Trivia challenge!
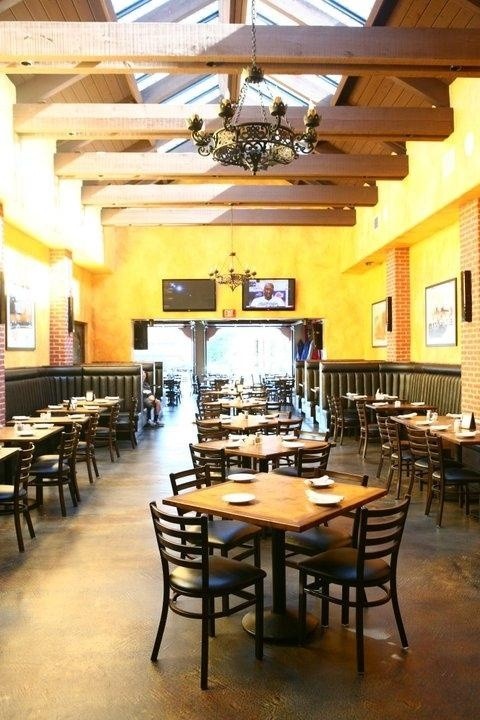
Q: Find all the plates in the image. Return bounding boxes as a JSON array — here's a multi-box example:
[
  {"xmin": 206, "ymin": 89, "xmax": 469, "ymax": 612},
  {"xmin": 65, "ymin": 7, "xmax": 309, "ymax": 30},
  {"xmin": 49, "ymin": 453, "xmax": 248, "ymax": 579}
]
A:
[
  {"xmin": 219, "ymin": 414, "xmax": 277, "ymax": 424},
  {"xmin": 12, "ymin": 415, "xmax": 30, "ymax": 420},
  {"xmin": 48, "ymin": 405, "xmax": 63, "ymax": 410},
  {"xmin": 69, "ymin": 413, "xmax": 86, "ymax": 419},
  {"xmin": 85, "ymin": 405, "xmax": 99, "ymax": 409},
  {"xmin": 398, "ymin": 412, "xmax": 476, "ymax": 439},
  {"xmin": 226, "ymin": 435, "xmax": 304, "ymax": 450},
  {"xmin": 410, "ymin": 402, "xmax": 426, "ymax": 405},
  {"xmin": 32, "ymin": 424, "xmax": 54, "ymax": 428},
  {"xmin": 373, "ymin": 402, "xmax": 388, "ymax": 406},
  {"xmin": 226, "ymin": 473, "xmax": 344, "ymax": 524}
]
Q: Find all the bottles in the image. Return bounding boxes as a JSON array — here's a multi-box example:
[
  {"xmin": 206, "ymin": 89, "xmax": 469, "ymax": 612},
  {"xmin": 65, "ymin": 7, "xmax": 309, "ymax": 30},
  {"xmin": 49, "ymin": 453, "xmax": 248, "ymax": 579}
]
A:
[
  {"xmin": 244, "ymin": 412, "xmax": 248, "ymax": 420},
  {"xmin": 40, "ymin": 413, "xmax": 46, "ymax": 421},
  {"xmin": 47, "ymin": 411, "xmax": 51, "ymax": 418},
  {"xmin": 256, "ymin": 433, "xmax": 261, "ymax": 444}
]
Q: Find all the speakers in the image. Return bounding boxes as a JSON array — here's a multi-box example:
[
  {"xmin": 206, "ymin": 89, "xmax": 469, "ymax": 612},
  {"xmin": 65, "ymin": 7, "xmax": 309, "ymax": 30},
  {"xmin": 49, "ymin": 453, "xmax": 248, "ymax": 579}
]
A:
[
  {"xmin": 133, "ymin": 321, "xmax": 148, "ymax": 350},
  {"xmin": 312, "ymin": 321, "xmax": 323, "ymax": 350}
]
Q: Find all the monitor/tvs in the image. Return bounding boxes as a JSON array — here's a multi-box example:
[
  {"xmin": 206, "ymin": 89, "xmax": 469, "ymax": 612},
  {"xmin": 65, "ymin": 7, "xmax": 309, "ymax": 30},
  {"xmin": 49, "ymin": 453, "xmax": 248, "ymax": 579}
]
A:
[
  {"xmin": 162, "ymin": 278, "xmax": 216, "ymax": 311},
  {"xmin": 242, "ymin": 278, "xmax": 296, "ymax": 311}
]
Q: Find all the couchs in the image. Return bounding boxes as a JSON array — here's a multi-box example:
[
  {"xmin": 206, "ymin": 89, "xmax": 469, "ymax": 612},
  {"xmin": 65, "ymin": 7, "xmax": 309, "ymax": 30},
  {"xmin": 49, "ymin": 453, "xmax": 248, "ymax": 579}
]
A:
[
  {"xmin": 320, "ymin": 360, "xmax": 461, "ymax": 434},
  {"xmin": 156, "ymin": 362, "xmax": 163, "ymax": 409},
  {"xmin": 5, "ymin": 366, "xmax": 141, "ymax": 440},
  {"xmin": 295, "ymin": 361, "xmax": 303, "ymax": 413},
  {"xmin": 144, "ymin": 363, "xmax": 154, "ymax": 426},
  {"xmin": 305, "ymin": 362, "xmax": 319, "ymax": 423}
]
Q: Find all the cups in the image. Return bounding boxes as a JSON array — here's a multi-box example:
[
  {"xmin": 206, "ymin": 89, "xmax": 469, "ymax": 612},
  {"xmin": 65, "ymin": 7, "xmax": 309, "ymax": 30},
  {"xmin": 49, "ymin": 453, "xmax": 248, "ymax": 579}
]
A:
[{"xmin": 395, "ymin": 401, "xmax": 401, "ymax": 408}]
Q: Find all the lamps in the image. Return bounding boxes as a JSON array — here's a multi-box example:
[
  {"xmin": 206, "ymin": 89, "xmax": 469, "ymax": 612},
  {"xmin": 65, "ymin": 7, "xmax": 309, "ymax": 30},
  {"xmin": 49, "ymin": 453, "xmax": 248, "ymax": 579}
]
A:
[
  {"xmin": 187, "ymin": 1, "xmax": 323, "ymax": 178},
  {"xmin": 209, "ymin": 204, "xmax": 261, "ymax": 291}
]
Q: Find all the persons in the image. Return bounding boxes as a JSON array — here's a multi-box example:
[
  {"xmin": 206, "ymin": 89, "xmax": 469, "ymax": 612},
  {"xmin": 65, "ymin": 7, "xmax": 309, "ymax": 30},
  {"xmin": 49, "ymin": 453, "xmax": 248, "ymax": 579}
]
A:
[
  {"xmin": 140, "ymin": 370, "xmax": 165, "ymax": 428},
  {"xmin": 250, "ymin": 283, "xmax": 285, "ymax": 308}
]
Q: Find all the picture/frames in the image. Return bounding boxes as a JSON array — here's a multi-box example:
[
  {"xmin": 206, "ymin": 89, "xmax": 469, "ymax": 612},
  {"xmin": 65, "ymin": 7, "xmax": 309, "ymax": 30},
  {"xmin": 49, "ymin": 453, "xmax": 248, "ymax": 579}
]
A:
[
  {"xmin": 372, "ymin": 300, "xmax": 388, "ymax": 348},
  {"xmin": 424, "ymin": 278, "xmax": 458, "ymax": 349},
  {"xmin": 5, "ymin": 278, "xmax": 36, "ymax": 352}
]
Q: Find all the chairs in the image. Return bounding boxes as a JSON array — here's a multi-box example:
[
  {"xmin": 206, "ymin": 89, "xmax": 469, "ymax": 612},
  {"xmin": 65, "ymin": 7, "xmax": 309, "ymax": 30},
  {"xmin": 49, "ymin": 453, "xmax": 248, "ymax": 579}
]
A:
[
  {"xmin": 297, "ymin": 443, "xmax": 331, "ymax": 527},
  {"xmin": 285, "ymin": 468, "xmax": 369, "ymax": 627},
  {"xmin": 169, "ymin": 464, "xmax": 262, "ymax": 568},
  {"xmin": 57, "ymin": 414, "xmax": 100, "ymax": 483},
  {"xmin": 92, "ymin": 402, "xmax": 120, "ymax": 462},
  {"xmin": 21, "ymin": 428, "xmax": 78, "ymax": 516},
  {"xmin": 112, "ymin": 394, "xmax": 138, "ymax": 449},
  {"xmin": 328, "ymin": 395, "xmax": 480, "ymax": 528},
  {"xmin": 298, "ymin": 495, "xmax": 411, "ymax": 675},
  {"xmin": 189, "ymin": 443, "xmax": 226, "ymax": 516},
  {"xmin": 149, "ymin": 501, "xmax": 266, "ymax": 691},
  {"xmin": 195, "ymin": 371, "xmax": 302, "ymax": 439},
  {"xmin": 0, "ymin": 442, "xmax": 35, "ymax": 553}
]
